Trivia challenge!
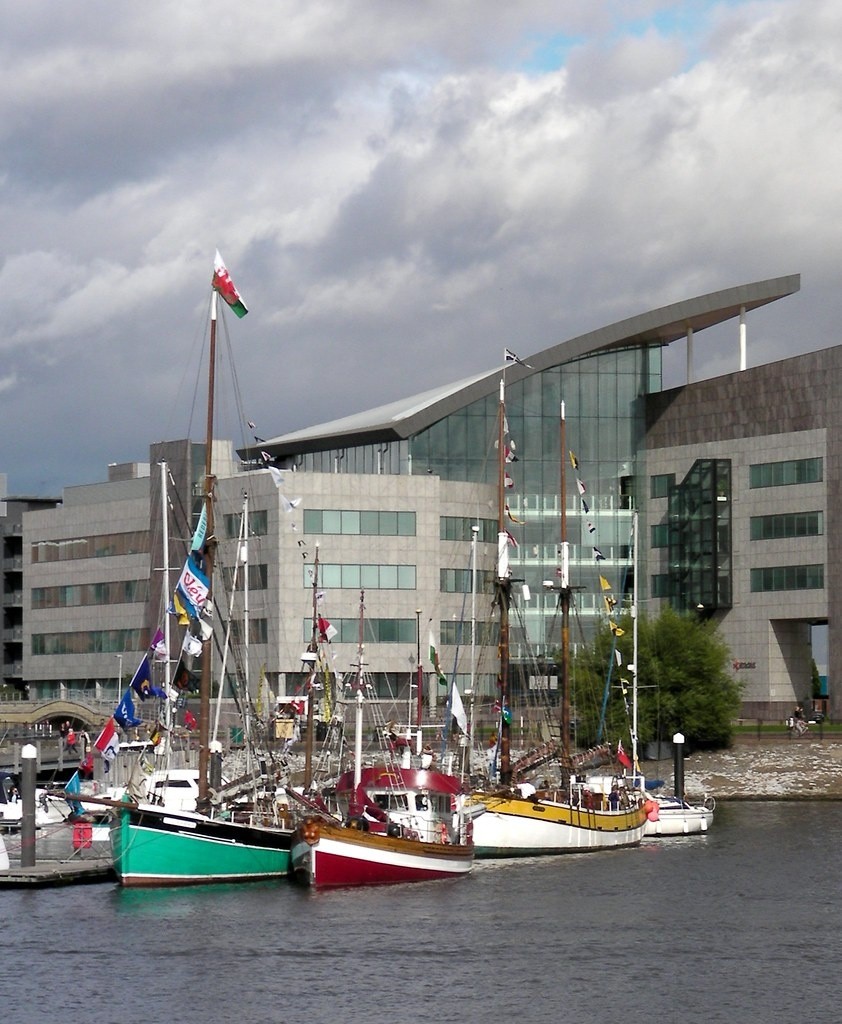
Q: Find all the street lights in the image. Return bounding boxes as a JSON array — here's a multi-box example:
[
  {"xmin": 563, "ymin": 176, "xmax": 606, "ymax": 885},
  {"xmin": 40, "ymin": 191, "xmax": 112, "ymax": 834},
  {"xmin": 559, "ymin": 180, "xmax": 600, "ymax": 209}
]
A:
[
  {"xmin": 114, "ymin": 654, "xmax": 123, "ymax": 704},
  {"xmin": 415, "ymin": 609, "xmax": 423, "ymax": 666}
]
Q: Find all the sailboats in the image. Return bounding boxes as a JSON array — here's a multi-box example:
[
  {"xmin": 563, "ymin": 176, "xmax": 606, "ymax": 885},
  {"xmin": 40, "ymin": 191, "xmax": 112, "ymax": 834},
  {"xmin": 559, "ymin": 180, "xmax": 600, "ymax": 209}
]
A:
[{"xmin": 0, "ymin": 249, "xmax": 715, "ymax": 887}]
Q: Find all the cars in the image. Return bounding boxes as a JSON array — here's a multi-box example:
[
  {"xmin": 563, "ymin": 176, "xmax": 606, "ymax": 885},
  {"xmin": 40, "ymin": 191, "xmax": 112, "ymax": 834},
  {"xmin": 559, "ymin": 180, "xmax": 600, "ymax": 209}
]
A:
[{"xmin": 811, "ymin": 712, "xmax": 825, "ymax": 724}]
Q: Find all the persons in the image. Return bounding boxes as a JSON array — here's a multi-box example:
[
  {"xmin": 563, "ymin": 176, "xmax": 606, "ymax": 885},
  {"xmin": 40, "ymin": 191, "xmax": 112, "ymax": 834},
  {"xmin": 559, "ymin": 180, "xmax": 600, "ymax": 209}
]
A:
[
  {"xmin": 514, "ymin": 778, "xmax": 536, "ymax": 800},
  {"xmin": 793, "ymin": 706, "xmax": 804, "ymax": 721},
  {"xmin": 9, "ymin": 786, "xmax": 19, "ymax": 804},
  {"xmin": 608, "ymin": 785, "xmax": 629, "ymax": 811},
  {"xmin": 100, "ymin": 719, "xmax": 105, "ymax": 726},
  {"xmin": 61, "ymin": 721, "xmax": 91, "ymax": 755},
  {"xmin": 539, "ymin": 780, "xmax": 550, "ymax": 789}
]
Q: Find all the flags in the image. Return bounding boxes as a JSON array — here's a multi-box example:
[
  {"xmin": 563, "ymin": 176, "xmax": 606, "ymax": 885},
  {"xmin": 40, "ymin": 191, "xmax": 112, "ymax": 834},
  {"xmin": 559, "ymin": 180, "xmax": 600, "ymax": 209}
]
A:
[
  {"xmin": 181, "ymin": 629, "xmax": 203, "ymax": 657},
  {"xmin": 62, "ymin": 771, "xmax": 86, "ymax": 819},
  {"xmin": 171, "ymin": 555, "xmax": 212, "ymax": 624},
  {"xmin": 150, "ymin": 730, "xmax": 161, "ymax": 746},
  {"xmin": 173, "ymin": 657, "xmax": 202, "ymax": 693},
  {"xmin": 445, "ymin": 681, "xmax": 468, "ymax": 737},
  {"xmin": 112, "ymin": 689, "xmax": 144, "ymax": 731},
  {"xmin": 130, "ymin": 657, "xmax": 168, "ymax": 703},
  {"xmin": 429, "ymin": 629, "xmax": 448, "ymax": 687},
  {"xmin": 150, "ymin": 629, "xmax": 166, "ymax": 656},
  {"xmin": 190, "ymin": 615, "xmax": 214, "ymax": 640},
  {"xmin": 184, "ymin": 709, "xmax": 197, "ymax": 731},
  {"xmin": 95, "ymin": 717, "xmax": 120, "ymax": 763},
  {"xmin": 318, "ymin": 616, "xmax": 338, "ymax": 642},
  {"xmin": 618, "ymin": 742, "xmax": 631, "ymax": 772},
  {"xmin": 212, "ymin": 248, "xmax": 248, "ymax": 319}
]
